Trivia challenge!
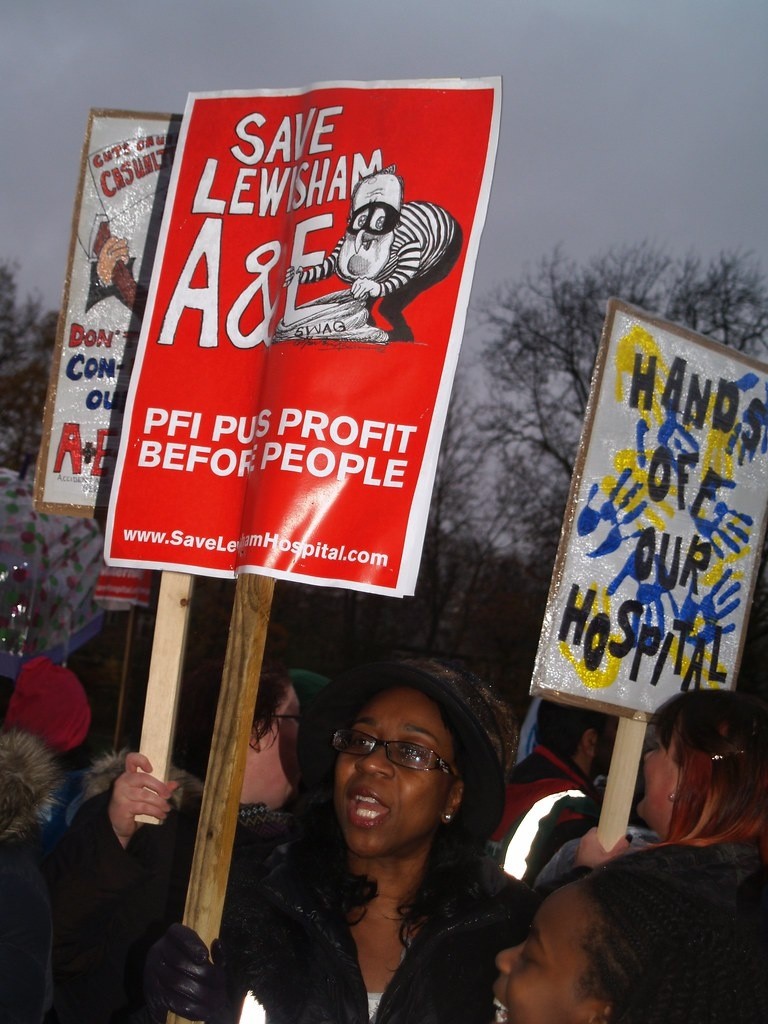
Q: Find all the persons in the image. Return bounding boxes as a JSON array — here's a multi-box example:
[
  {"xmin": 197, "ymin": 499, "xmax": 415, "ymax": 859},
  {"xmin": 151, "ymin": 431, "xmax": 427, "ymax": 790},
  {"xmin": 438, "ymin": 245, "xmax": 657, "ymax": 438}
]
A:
[{"xmin": 0, "ymin": 623, "xmax": 768, "ymax": 1024}]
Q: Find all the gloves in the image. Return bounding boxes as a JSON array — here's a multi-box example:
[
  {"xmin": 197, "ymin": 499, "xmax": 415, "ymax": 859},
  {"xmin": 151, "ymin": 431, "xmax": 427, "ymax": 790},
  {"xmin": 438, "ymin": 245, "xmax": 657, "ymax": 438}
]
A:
[{"xmin": 144, "ymin": 924, "xmax": 236, "ymax": 1024}]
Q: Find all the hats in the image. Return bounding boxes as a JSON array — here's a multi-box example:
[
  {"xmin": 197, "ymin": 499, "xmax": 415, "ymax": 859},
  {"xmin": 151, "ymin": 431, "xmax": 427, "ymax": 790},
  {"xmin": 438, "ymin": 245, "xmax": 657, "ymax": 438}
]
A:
[
  {"xmin": 297, "ymin": 657, "xmax": 518, "ymax": 837},
  {"xmin": 4, "ymin": 657, "xmax": 91, "ymax": 752},
  {"xmin": 289, "ymin": 668, "xmax": 333, "ymax": 714}
]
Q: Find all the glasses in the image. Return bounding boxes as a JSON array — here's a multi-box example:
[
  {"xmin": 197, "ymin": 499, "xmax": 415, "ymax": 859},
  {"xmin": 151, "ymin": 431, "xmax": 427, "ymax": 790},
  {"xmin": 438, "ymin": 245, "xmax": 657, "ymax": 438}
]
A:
[{"xmin": 333, "ymin": 728, "xmax": 459, "ymax": 778}]
